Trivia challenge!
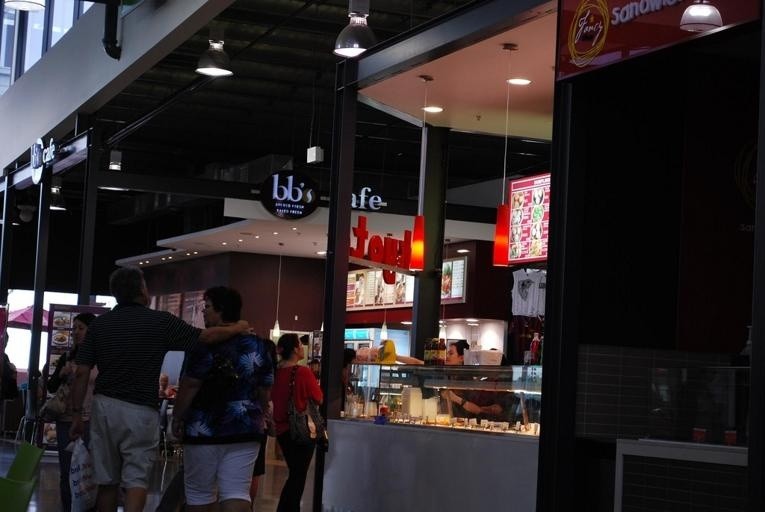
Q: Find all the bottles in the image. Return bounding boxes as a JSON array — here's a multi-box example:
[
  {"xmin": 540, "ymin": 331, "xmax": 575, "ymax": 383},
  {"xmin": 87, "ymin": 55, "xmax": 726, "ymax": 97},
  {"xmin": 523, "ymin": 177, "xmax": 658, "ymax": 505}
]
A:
[
  {"xmin": 530, "ymin": 332, "xmax": 544, "ymax": 365},
  {"xmin": 423, "ymin": 337, "xmax": 446, "ymax": 364}
]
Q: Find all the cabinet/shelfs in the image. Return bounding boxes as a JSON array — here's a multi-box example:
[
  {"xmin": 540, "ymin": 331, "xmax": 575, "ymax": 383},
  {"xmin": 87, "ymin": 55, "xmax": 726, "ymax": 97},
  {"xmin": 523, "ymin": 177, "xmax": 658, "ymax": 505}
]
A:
[{"xmin": 321, "ymin": 363, "xmax": 542, "ymax": 511}]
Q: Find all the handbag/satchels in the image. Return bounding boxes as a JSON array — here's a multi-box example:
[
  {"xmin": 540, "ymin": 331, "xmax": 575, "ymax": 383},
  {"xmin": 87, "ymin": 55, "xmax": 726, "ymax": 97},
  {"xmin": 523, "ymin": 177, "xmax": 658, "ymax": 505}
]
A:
[
  {"xmin": 38, "ymin": 386, "xmax": 70, "ymax": 424},
  {"xmin": 287, "ymin": 365, "xmax": 329, "ymax": 452}
]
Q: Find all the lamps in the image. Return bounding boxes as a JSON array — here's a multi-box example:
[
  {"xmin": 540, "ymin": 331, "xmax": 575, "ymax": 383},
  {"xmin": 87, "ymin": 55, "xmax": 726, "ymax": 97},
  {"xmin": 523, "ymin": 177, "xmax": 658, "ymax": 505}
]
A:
[
  {"xmin": 272, "ymin": 243, "xmax": 284, "ymax": 338},
  {"xmin": 409, "ymin": 72, "xmax": 434, "ymax": 271},
  {"xmin": 680, "ymin": 0, "xmax": 723, "ymax": 33},
  {"xmin": 332, "ymin": 0, "xmax": 376, "ymax": 58},
  {"xmin": 492, "ymin": 42, "xmax": 519, "ymax": 268},
  {"xmin": 194, "ymin": 27, "xmax": 235, "ymax": 78},
  {"xmin": 17, "ymin": 205, "xmax": 37, "ymax": 222},
  {"xmin": 109, "ymin": 136, "xmax": 123, "ymax": 171},
  {"xmin": 49, "ymin": 175, "xmax": 67, "ymax": 211}
]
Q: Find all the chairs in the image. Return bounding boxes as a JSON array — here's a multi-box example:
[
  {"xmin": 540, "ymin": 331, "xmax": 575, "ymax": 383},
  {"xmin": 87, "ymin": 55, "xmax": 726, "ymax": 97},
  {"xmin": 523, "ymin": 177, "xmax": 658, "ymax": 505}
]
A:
[{"xmin": 0, "ymin": 388, "xmax": 183, "ymax": 512}]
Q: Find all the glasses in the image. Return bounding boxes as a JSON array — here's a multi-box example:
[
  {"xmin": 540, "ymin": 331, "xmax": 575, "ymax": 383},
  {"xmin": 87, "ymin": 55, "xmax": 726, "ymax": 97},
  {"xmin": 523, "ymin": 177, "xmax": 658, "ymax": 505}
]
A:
[{"xmin": 202, "ymin": 303, "xmax": 214, "ymax": 310}]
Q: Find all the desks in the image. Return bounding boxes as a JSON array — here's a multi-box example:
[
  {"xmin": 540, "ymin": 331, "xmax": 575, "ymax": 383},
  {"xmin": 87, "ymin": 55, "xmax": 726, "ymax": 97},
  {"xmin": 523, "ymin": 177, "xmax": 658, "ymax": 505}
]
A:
[{"xmin": 614, "ymin": 436, "xmax": 749, "ymax": 511}]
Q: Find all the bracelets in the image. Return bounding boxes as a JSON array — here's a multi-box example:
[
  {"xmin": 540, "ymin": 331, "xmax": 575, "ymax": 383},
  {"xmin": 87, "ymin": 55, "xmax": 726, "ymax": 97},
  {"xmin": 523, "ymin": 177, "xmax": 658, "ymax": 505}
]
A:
[
  {"xmin": 459, "ymin": 398, "xmax": 468, "ymax": 407},
  {"xmin": 72, "ymin": 406, "xmax": 84, "ymax": 414}
]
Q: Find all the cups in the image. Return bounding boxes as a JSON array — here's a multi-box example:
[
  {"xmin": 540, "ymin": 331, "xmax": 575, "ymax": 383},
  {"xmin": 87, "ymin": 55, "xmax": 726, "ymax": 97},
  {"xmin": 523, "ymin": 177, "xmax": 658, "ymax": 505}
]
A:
[
  {"xmin": 524, "ymin": 350, "xmax": 530, "ymax": 363},
  {"xmin": 469, "ymin": 334, "xmax": 482, "ymax": 366}
]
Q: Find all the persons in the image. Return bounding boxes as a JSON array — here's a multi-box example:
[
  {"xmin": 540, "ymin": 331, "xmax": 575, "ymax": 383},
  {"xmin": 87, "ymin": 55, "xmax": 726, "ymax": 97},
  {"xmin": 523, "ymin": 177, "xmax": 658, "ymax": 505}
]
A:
[
  {"xmin": 64, "ymin": 263, "xmax": 256, "ymax": 511},
  {"xmin": 157, "ymin": 371, "xmax": 171, "ymax": 409},
  {"xmin": 342, "ymin": 346, "xmax": 366, "ymax": 418},
  {"xmin": 247, "ymin": 429, "xmax": 269, "ymax": 511},
  {"xmin": 267, "ymin": 332, "xmax": 325, "ymax": 512},
  {"xmin": 463, "ymin": 347, "xmax": 514, "ymax": 422},
  {"xmin": 0, "ymin": 332, "xmax": 18, "ymax": 446},
  {"xmin": 170, "ymin": 283, "xmax": 277, "ymax": 511},
  {"xmin": 307, "ymin": 342, "xmax": 322, "ymax": 362},
  {"xmin": 17, "ymin": 368, "xmax": 41, "ymax": 401},
  {"xmin": 48, "ymin": 311, "xmax": 97, "ymax": 512},
  {"xmin": 393, "ymin": 339, "xmax": 482, "ymax": 419}
]
